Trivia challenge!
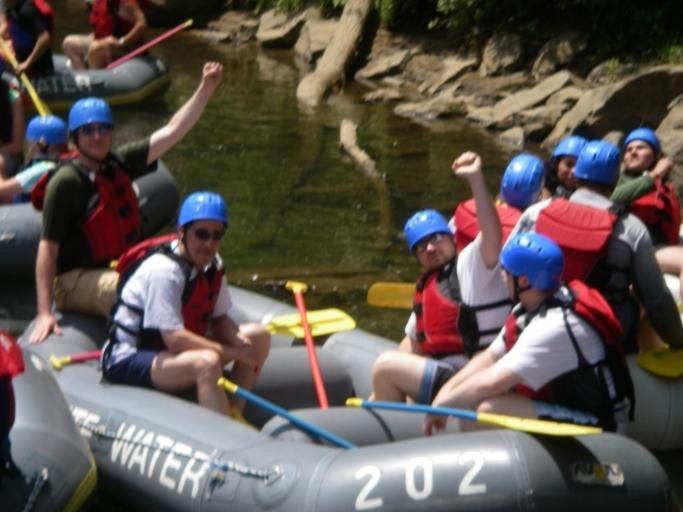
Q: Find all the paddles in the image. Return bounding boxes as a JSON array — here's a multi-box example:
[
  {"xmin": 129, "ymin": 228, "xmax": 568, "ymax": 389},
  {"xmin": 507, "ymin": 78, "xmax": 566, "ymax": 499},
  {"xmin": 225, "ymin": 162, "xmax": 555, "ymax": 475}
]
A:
[
  {"xmin": 50, "ymin": 308, "xmax": 356, "ymax": 370},
  {"xmin": 638, "ymin": 347, "xmax": 683, "ymax": 379},
  {"xmin": 347, "ymin": 396, "xmax": 604, "ymax": 436},
  {"xmin": 365, "ymin": 282, "xmax": 417, "ymax": 309},
  {"xmin": 2, "ymin": 37, "xmax": 53, "ymax": 118}
]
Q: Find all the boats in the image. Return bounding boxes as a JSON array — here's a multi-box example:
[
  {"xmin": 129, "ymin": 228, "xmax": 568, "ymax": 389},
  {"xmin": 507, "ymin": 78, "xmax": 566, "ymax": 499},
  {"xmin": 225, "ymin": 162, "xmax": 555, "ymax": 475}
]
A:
[
  {"xmin": 592, "ymin": 269, "xmax": 682, "ymax": 456},
  {"xmin": 0, "ymin": 333, "xmax": 98, "ymax": 511},
  {"xmin": 21, "ymin": 282, "xmax": 670, "ymax": 512},
  {"xmin": 0, "ymin": 152, "xmax": 180, "ymax": 271},
  {"xmin": 0, "ymin": 53, "xmax": 170, "ymax": 111}
]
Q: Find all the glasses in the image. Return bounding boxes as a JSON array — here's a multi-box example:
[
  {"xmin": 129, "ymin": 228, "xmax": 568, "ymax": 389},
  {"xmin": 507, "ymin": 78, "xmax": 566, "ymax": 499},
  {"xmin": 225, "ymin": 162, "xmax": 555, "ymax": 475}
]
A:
[
  {"xmin": 412, "ymin": 232, "xmax": 444, "ymax": 255},
  {"xmin": 80, "ymin": 124, "xmax": 112, "ymax": 136},
  {"xmin": 187, "ymin": 227, "xmax": 224, "ymax": 241}
]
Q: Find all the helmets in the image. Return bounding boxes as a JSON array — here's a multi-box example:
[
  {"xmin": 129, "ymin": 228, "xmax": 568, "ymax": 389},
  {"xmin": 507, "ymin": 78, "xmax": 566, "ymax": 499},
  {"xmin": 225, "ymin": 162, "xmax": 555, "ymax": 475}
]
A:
[
  {"xmin": 499, "ymin": 152, "xmax": 548, "ymax": 210},
  {"xmin": 66, "ymin": 95, "xmax": 114, "ymax": 136},
  {"xmin": 622, "ymin": 126, "xmax": 661, "ymax": 158},
  {"xmin": 550, "ymin": 133, "xmax": 590, "ymax": 163},
  {"xmin": 497, "ymin": 230, "xmax": 565, "ymax": 293},
  {"xmin": 403, "ymin": 207, "xmax": 454, "ymax": 256},
  {"xmin": 572, "ymin": 139, "xmax": 624, "ymax": 186},
  {"xmin": 24, "ymin": 114, "xmax": 69, "ymax": 146},
  {"xmin": 175, "ymin": 190, "xmax": 229, "ymax": 231}
]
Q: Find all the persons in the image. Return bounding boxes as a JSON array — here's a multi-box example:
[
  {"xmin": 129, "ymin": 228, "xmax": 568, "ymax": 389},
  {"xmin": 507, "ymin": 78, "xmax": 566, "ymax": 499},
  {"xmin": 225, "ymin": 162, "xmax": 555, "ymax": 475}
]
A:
[
  {"xmin": 24, "ymin": 59, "xmax": 227, "ymax": 345},
  {"xmin": 97, "ymin": 190, "xmax": 274, "ymax": 420},
  {"xmin": 0, "ymin": 0, "xmax": 146, "ymax": 202},
  {"xmin": 367, "ymin": 123, "xmax": 683, "ymax": 435}
]
